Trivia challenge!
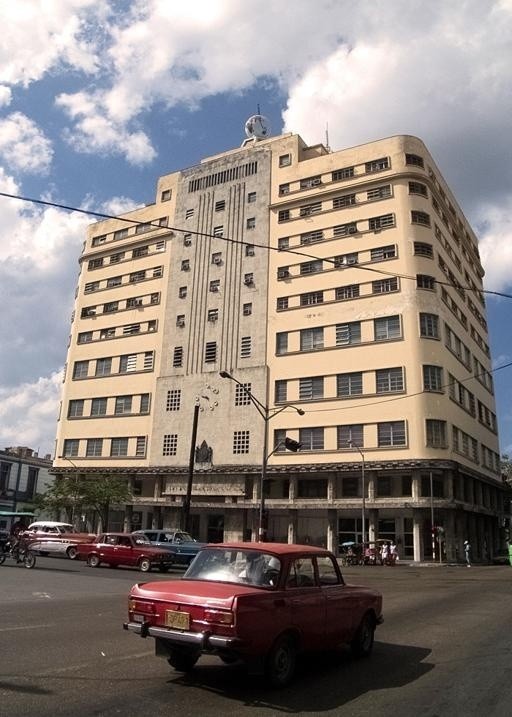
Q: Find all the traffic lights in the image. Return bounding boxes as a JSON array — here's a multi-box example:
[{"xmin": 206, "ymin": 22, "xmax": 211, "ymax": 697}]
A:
[{"xmin": 286, "ymin": 437, "xmax": 301, "ymax": 453}]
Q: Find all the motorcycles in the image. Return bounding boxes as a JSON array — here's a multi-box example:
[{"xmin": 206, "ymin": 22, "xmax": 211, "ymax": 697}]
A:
[{"xmin": 0, "ymin": 533, "xmax": 37, "ymax": 568}]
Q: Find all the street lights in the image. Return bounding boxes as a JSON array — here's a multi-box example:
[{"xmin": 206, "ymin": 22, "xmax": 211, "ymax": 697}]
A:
[
  {"xmin": 219, "ymin": 372, "xmax": 303, "ymax": 538},
  {"xmin": 348, "ymin": 438, "xmax": 366, "ymax": 543},
  {"xmin": 57, "ymin": 455, "xmax": 78, "ymax": 526}
]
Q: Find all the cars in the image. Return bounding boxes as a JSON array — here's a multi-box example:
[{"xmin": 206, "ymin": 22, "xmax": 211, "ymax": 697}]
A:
[
  {"xmin": 131, "ymin": 530, "xmax": 207, "ymax": 567},
  {"xmin": 23, "ymin": 521, "xmax": 85, "ymax": 560},
  {"xmin": 77, "ymin": 533, "xmax": 178, "ymax": 572},
  {"xmin": 123, "ymin": 541, "xmax": 386, "ymax": 693}
]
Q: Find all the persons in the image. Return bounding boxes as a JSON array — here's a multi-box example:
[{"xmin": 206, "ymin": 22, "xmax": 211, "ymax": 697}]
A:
[
  {"xmin": 9, "ymin": 516, "xmax": 28, "ymax": 563},
  {"xmin": 379, "ymin": 540, "xmax": 398, "ymax": 567},
  {"xmin": 238, "ymin": 553, "xmax": 275, "ymax": 585},
  {"xmin": 464, "ymin": 540, "xmax": 472, "ymax": 567}
]
